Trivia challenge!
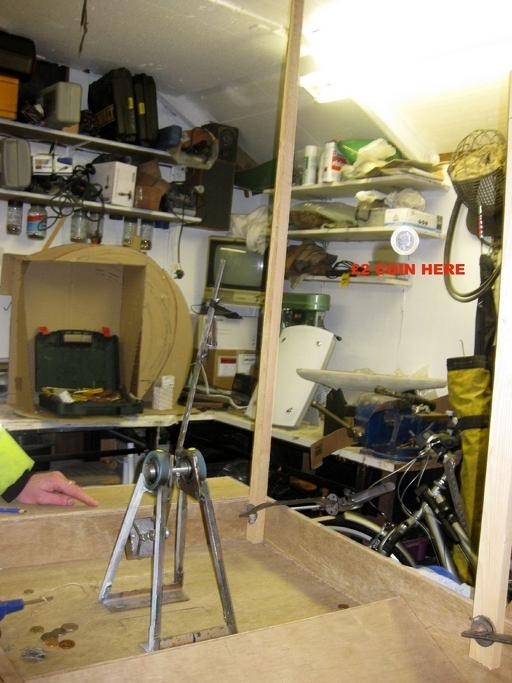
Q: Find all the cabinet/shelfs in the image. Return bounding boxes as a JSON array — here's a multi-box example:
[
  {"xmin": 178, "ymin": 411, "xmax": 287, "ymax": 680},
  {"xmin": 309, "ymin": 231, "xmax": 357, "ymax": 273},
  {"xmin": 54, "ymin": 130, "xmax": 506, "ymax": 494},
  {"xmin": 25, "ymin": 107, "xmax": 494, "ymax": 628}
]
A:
[
  {"xmin": 1, "ymin": 116, "xmax": 208, "ymax": 229},
  {"xmin": 261, "ymin": 172, "xmax": 449, "ymax": 291}
]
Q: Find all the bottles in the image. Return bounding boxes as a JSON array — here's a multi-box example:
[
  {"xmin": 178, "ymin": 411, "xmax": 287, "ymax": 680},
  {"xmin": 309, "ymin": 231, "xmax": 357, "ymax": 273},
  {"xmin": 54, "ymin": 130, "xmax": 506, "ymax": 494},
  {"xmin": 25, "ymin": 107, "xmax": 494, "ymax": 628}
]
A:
[
  {"xmin": 87, "ymin": 210, "xmax": 104, "ymax": 240},
  {"xmin": 69, "ymin": 206, "xmax": 89, "ymax": 243},
  {"xmin": 26, "ymin": 202, "xmax": 48, "ymax": 240},
  {"xmin": 6, "ymin": 198, "xmax": 24, "ymax": 236},
  {"xmin": 302, "ymin": 144, "xmax": 318, "ymax": 186},
  {"xmin": 122, "ymin": 215, "xmax": 138, "ymax": 247},
  {"xmin": 139, "ymin": 218, "xmax": 155, "ymax": 251}
]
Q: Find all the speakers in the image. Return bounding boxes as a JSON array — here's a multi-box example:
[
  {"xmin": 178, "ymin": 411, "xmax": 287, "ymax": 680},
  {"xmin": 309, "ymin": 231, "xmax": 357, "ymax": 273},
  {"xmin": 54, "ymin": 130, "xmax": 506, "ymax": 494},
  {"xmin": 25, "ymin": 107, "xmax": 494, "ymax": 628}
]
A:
[
  {"xmin": 186, "ymin": 157, "xmax": 238, "ymax": 230},
  {"xmin": 201, "ymin": 123, "xmax": 238, "ymax": 161}
]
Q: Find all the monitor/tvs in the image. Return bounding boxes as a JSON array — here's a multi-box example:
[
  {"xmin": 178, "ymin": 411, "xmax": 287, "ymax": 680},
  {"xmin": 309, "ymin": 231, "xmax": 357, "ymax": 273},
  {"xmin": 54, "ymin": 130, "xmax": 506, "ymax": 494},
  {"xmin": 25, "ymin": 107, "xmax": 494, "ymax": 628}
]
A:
[{"xmin": 204, "ymin": 236, "xmax": 267, "ymax": 306}]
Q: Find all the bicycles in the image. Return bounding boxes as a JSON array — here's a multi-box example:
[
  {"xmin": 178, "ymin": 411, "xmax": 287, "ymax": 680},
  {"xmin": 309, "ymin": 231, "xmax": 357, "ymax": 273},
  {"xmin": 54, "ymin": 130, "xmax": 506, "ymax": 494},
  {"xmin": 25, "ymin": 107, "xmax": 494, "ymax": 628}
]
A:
[{"xmin": 309, "ymin": 409, "xmax": 479, "ymax": 578}]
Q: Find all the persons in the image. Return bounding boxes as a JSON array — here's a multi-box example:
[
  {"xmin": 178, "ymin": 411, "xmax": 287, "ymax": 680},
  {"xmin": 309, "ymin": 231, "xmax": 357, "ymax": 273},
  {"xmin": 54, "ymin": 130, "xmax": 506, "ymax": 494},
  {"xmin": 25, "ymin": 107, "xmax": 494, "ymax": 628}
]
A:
[{"xmin": 0, "ymin": 425, "xmax": 99, "ymax": 508}]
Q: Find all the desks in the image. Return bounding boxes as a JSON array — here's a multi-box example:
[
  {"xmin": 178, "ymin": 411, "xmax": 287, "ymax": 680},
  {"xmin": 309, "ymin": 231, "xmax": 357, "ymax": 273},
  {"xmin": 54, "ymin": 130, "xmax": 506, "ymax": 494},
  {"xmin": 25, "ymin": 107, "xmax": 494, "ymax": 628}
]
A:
[{"xmin": 0, "ymin": 394, "xmax": 512, "ymax": 683}]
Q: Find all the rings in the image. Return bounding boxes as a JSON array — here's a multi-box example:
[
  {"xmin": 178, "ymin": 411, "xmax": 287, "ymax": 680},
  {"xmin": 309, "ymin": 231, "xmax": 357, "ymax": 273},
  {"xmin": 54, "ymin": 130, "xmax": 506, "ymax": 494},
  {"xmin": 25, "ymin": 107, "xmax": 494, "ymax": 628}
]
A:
[{"xmin": 66, "ymin": 478, "xmax": 76, "ymax": 486}]
